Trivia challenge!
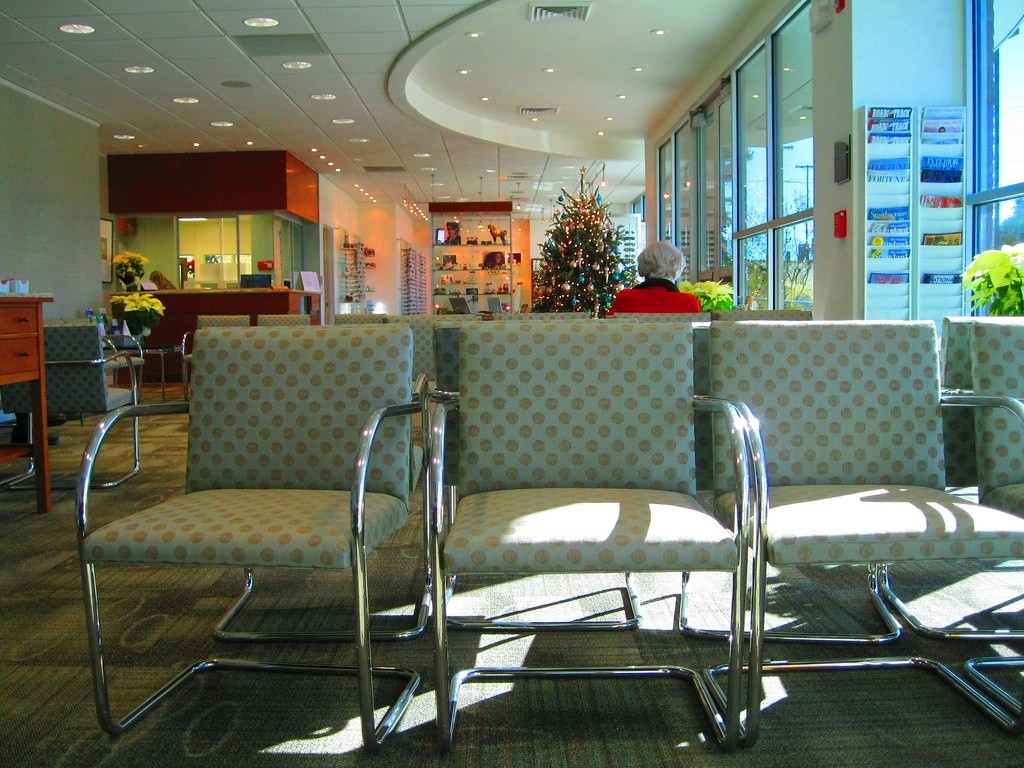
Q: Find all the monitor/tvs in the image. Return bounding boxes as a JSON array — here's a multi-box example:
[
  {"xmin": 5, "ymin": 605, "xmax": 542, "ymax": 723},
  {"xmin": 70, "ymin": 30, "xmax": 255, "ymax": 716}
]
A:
[{"xmin": 240, "ymin": 274, "xmax": 272, "ymax": 288}]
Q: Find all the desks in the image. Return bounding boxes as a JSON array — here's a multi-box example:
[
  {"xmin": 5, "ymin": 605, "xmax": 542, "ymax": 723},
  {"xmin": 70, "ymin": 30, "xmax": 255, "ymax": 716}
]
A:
[
  {"xmin": 0, "ymin": 297, "xmax": 54, "ymax": 514},
  {"xmin": 103, "ymin": 345, "xmax": 182, "ymax": 402}
]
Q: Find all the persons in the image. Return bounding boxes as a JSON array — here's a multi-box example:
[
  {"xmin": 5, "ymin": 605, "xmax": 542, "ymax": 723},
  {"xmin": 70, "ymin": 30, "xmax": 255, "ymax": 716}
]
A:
[
  {"xmin": 445, "ymin": 221, "xmax": 461, "ymax": 245},
  {"xmin": 483, "ymin": 252, "xmax": 504, "ymax": 268},
  {"xmin": 605, "ymin": 240, "xmax": 701, "ymax": 316},
  {"xmin": 150, "ymin": 270, "xmax": 177, "ymax": 291}
]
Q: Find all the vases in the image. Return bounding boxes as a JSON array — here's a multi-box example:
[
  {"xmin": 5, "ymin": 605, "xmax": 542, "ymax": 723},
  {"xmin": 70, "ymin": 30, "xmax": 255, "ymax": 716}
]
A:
[
  {"xmin": 124, "ymin": 336, "xmax": 140, "ymax": 349},
  {"xmin": 125, "ymin": 275, "xmax": 136, "ymax": 290}
]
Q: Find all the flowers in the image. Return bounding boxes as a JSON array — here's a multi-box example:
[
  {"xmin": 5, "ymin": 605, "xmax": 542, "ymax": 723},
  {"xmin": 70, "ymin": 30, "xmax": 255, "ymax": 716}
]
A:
[
  {"xmin": 109, "ymin": 292, "xmax": 166, "ymax": 336},
  {"xmin": 113, "ymin": 251, "xmax": 149, "ymax": 285}
]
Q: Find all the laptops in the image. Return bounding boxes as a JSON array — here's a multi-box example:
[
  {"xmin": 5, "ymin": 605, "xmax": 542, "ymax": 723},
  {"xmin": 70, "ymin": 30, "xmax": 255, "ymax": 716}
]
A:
[
  {"xmin": 487, "ymin": 296, "xmax": 508, "ymax": 313},
  {"xmin": 449, "ymin": 297, "xmax": 485, "ymax": 315}
]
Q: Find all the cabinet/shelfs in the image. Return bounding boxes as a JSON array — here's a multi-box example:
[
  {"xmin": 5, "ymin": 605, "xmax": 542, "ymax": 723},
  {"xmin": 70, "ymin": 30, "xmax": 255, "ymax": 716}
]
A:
[{"xmin": 428, "ymin": 201, "xmax": 512, "ymax": 315}]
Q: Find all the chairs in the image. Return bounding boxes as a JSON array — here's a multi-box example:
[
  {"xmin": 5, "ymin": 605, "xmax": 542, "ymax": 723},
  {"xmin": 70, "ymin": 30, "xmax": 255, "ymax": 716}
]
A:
[
  {"xmin": 705, "ymin": 319, "xmax": 1024, "ymax": 748},
  {"xmin": 965, "ymin": 320, "xmax": 1024, "ymax": 717},
  {"xmin": 75, "ymin": 324, "xmax": 428, "ymax": 750},
  {"xmin": 426, "ymin": 320, "xmax": 750, "ymax": 753},
  {"xmin": 0, "ymin": 320, "xmax": 144, "ymax": 491},
  {"xmin": 181, "ymin": 313, "xmax": 979, "ymax": 643},
  {"xmin": 879, "ymin": 316, "xmax": 1024, "ymax": 641}
]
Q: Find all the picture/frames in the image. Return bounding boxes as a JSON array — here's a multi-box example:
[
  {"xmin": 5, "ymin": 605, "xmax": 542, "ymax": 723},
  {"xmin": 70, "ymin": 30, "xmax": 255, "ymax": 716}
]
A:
[{"xmin": 100, "ymin": 217, "xmax": 114, "ymax": 284}]
